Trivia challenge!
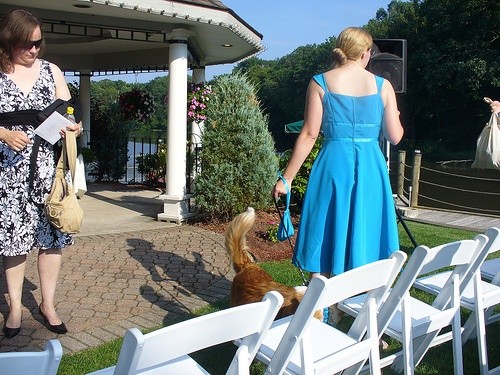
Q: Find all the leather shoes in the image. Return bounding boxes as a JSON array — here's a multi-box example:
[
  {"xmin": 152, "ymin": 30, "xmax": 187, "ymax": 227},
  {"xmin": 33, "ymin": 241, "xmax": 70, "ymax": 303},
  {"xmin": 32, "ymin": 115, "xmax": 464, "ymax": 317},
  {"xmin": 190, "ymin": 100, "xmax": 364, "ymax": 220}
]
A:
[
  {"xmin": 39, "ymin": 303, "xmax": 68, "ymax": 334},
  {"xmin": 4, "ymin": 307, "xmax": 23, "ymax": 339}
]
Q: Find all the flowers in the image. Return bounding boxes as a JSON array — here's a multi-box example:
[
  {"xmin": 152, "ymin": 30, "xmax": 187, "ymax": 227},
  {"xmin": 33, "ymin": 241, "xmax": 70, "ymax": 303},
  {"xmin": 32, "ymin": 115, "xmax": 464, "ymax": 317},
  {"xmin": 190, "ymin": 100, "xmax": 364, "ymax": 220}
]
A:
[
  {"xmin": 187, "ymin": 80, "xmax": 212, "ymax": 123},
  {"xmin": 116, "ymin": 86, "xmax": 152, "ymax": 122}
]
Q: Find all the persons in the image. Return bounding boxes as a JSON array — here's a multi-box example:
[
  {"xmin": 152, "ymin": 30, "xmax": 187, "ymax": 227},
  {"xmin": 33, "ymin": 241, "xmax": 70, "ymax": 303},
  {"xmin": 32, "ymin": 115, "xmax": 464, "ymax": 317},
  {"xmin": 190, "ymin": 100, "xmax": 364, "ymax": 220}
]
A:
[
  {"xmin": 0, "ymin": 9, "xmax": 83, "ymax": 339},
  {"xmin": 270, "ymin": 26, "xmax": 405, "ymax": 351},
  {"xmin": 489, "ymin": 101, "xmax": 500, "ymax": 114}
]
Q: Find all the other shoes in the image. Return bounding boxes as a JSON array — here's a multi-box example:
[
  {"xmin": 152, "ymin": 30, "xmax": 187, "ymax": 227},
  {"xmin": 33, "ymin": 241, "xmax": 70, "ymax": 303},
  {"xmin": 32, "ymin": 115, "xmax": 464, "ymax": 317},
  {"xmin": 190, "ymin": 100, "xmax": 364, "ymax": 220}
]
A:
[
  {"xmin": 328, "ymin": 306, "xmax": 341, "ymax": 327},
  {"xmin": 379, "ymin": 339, "xmax": 389, "ymax": 351}
]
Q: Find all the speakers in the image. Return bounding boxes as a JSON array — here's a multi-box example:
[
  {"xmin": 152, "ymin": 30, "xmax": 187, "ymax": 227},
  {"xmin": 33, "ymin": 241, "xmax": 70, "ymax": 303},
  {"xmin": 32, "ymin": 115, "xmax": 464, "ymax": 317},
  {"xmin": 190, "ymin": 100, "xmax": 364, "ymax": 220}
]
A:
[{"xmin": 365, "ymin": 39, "xmax": 407, "ymax": 94}]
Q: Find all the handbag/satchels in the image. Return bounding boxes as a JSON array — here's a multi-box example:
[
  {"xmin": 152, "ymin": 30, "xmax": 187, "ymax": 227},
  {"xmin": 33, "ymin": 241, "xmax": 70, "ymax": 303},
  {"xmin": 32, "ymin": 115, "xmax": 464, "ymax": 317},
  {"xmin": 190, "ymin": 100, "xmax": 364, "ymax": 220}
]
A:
[
  {"xmin": 471, "ymin": 97, "xmax": 500, "ymax": 170},
  {"xmin": 277, "ymin": 175, "xmax": 294, "ymax": 241},
  {"xmin": 45, "ymin": 130, "xmax": 83, "ymax": 234}
]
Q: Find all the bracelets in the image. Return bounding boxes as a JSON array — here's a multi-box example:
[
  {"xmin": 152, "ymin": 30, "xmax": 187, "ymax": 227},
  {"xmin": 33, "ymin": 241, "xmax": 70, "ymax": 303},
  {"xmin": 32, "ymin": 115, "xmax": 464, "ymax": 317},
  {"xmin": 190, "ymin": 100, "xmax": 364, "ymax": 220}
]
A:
[{"xmin": 282, "ymin": 176, "xmax": 295, "ymax": 180}]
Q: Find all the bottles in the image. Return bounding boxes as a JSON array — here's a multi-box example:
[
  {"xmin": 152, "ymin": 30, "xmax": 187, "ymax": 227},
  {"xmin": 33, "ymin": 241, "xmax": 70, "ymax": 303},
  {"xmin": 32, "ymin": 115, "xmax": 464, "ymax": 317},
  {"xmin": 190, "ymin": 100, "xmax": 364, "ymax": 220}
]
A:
[{"xmin": 63, "ymin": 107, "xmax": 75, "ymax": 123}]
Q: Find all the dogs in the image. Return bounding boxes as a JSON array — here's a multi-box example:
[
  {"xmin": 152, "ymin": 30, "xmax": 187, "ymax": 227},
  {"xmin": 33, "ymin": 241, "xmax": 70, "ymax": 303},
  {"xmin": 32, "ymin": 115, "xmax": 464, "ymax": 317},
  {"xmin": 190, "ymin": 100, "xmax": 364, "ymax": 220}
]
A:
[{"xmin": 222, "ymin": 205, "xmax": 324, "ymax": 324}]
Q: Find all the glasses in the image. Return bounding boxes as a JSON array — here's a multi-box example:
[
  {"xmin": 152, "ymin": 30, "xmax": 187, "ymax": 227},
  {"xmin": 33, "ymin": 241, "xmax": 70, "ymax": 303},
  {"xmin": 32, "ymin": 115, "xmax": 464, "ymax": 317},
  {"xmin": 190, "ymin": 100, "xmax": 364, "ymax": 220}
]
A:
[{"xmin": 28, "ymin": 37, "xmax": 43, "ymax": 50}]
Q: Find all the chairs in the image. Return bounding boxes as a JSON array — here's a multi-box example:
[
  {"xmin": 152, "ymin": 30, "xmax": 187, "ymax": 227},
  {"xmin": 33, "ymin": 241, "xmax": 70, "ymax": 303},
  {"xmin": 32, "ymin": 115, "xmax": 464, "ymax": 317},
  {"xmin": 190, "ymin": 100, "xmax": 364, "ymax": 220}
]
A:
[
  {"xmin": 471, "ymin": 257, "xmax": 500, "ymax": 338},
  {"xmin": 232, "ymin": 249, "xmax": 407, "ymax": 375},
  {"xmin": 85, "ymin": 292, "xmax": 284, "ymax": 375},
  {"xmin": 411, "ymin": 226, "xmax": 500, "ymax": 375},
  {"xmin": 337, "ymin": 234, "xmax": 489, "ymax": 375}
]
